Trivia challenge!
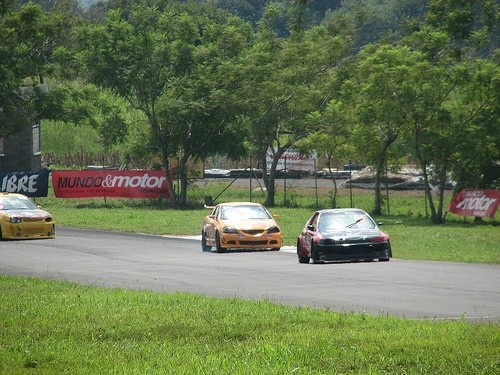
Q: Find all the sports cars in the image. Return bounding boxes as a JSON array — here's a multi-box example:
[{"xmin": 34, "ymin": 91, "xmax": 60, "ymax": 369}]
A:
[
  {"xmin": 201, "ymin": 201, "xmax": 283, "ymax": 253},
  {"xmin": 0, "ymin": 191, "xmax": 56, "ymax": 239},
  {"xmin": 297, "ymin": 208, "xmax": 393, "ymax": 264}
]
